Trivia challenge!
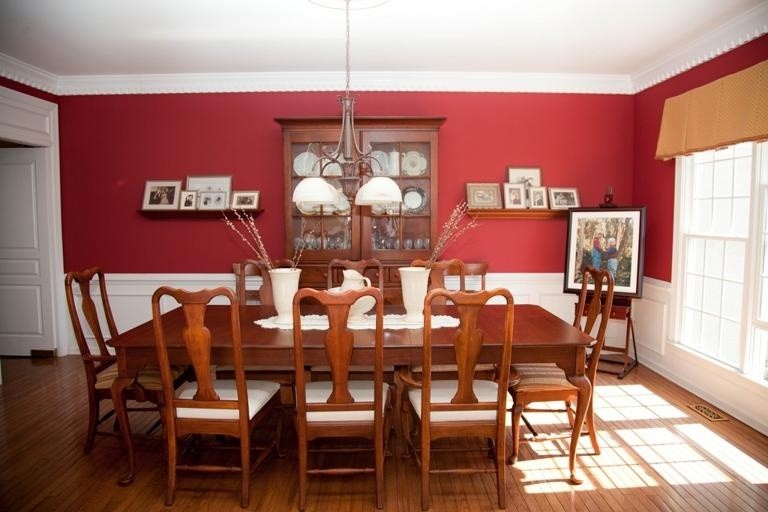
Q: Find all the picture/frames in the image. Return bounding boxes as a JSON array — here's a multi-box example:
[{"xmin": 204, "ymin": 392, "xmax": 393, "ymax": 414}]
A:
[
  {"xmin": 141, "ymin": 175, "xmax": 259, "ymax": 211},
  {"xmin": 467, "ymin": 166, "xmax": 581, "ymax": 211},
  {"xmin": 563, "ymin": 207, "xmax": 646, "ymax": 299}
]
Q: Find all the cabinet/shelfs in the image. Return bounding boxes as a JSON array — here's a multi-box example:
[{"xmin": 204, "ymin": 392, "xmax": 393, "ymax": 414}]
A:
[
  {"xmin": 274, "ymin": 115, "xmax": 447, "ymax": 306},
  {"xmin": 574, "ymin": 297, "xmax": 638, "ymax": 380}
]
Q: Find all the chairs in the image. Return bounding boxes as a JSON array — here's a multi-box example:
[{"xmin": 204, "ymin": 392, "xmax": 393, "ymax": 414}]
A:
[
  {"xmin": 402, "ymin": 287, "xmax": 515, "ymax": 511},
  {"xmin": 503, "ymin": 267, "xmax": 615, "ymax": 463},
  {"xmin": 411, "ymin": 259, "xmax": 466, "ymax": 306},
  {"xmin": 239, "ymin": 259, "xmax": 295, "ymax": 307},
  {"xmin": 64, "ymin": 267, "xmax": 189, "ymax": 455},
  {"xmin": 152, "ymin": 286, "xmax": 285, "ymax": 508},
  {"xmin": 326, "ymin": 256, "xmax": 384, "ymax": 310},
  {"xmin": 292, "ymin": 287, "xmax": 392, "ymax": 512}
]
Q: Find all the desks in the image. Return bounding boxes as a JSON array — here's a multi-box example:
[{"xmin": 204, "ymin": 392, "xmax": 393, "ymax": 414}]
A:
[{"xmin": 103, "ymin": 304, "xmax": 598, "ymax": 487}]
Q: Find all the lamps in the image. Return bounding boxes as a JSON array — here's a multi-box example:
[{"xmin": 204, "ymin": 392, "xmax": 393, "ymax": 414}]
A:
[{"xmin": 292, "ymin": 0, "xmax": 403, "ymax": 214}]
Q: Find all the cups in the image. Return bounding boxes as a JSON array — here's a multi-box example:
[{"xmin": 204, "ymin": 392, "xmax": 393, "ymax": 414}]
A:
[
  {"xmin": 295, "ymin": 234, "xmax": 344, "ymax": 249},
  {"xmin": 382, "ymin": 237, "xmax": 430, "ymax": 249}
]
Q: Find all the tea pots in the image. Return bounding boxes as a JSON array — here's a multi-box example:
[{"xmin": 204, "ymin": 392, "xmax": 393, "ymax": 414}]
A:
[{"xmin": 382, "ymin": 148, "xmax": 399, "ymax": 176}]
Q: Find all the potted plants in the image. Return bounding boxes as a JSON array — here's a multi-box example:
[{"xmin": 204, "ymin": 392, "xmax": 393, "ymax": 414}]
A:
[
  {"xmin": 398, "ymin": 267, "xmax": 431, "ymax": 322},
  {"xmin": 268, "ymin": 268, "xmax": 303, "ymax": 325}
]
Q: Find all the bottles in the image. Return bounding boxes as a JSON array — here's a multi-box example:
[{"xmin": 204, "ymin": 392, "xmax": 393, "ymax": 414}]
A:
[{"xmin": 371, "ymin": 226, "xmax": 380, "ymax": 250}]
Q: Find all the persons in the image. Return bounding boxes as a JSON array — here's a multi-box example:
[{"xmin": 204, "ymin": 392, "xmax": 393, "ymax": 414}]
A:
[
  {"xmin": 605, "ymin": 237, "xmax": 619, "ymax": 281},
  {"xmin": 534, "ymin": 192, "xmax": 543, "ymax": 206},
  {"xmin": 591, "ymin": 230, "xmax": 604, "ymax": 271},
  {"xmin": 154, "ymin": 190, "xmax": 169, "ymax": 205}
]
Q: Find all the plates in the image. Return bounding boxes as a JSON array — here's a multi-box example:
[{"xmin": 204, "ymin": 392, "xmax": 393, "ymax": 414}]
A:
[
  {"xmin": 371, "ymin": 151, "xmax": 390, "ymax": 175},
  {"xmin": 293, "ymin": 152, "xmax": 319, "ymax": 176},
  {"xmin": 323, "ymin": 152, "xmax": 345, "ymax": 178}
]
